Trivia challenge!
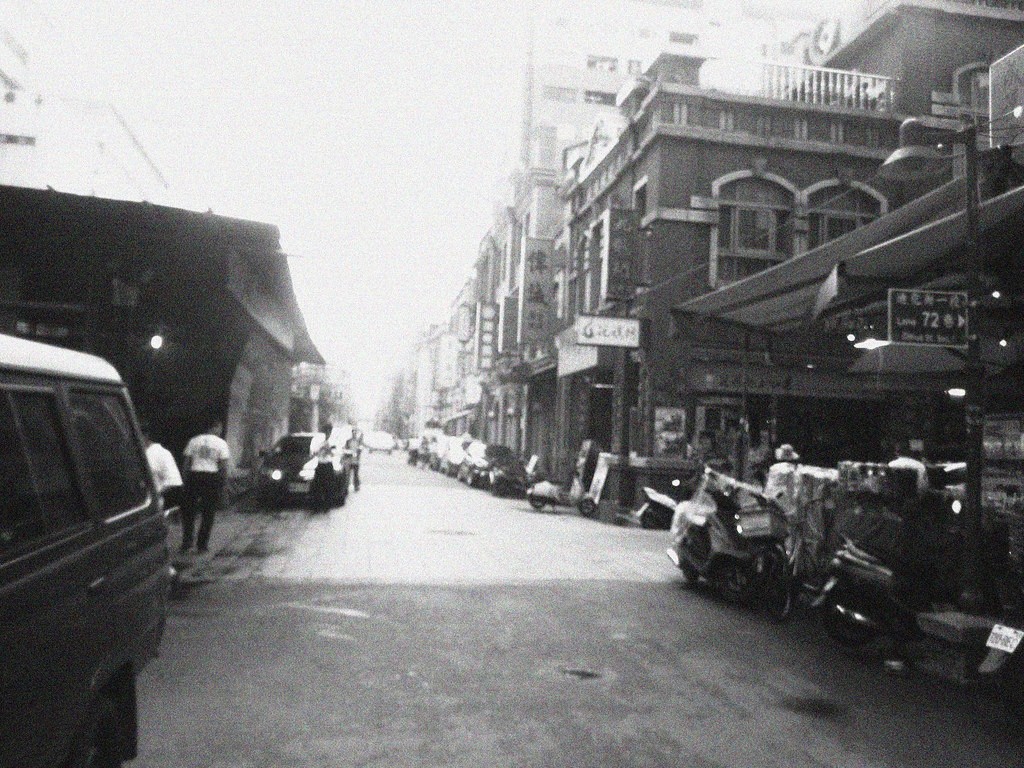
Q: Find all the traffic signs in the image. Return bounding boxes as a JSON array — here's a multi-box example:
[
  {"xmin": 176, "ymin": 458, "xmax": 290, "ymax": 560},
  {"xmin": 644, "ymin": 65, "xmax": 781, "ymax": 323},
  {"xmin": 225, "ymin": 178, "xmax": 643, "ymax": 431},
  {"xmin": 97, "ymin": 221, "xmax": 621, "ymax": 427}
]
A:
[{"xmin": 886, "ymin": 289, "xmax": 969, "ymax": 349}]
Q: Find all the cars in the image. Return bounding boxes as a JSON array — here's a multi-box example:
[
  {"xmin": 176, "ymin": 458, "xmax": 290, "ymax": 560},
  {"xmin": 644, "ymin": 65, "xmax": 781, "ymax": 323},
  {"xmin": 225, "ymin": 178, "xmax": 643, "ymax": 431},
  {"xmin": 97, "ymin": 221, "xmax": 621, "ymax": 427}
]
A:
[
  {"xmin": 368, "ymin": 432, "xmax": 393, "ymax": 454},
  {"xmin": 257, "ymin": 424, "xmax": 355, "ymax": 506},
  {"xmin": 418, "ymin": 435, "xmax": 536, "ymax": 498}
]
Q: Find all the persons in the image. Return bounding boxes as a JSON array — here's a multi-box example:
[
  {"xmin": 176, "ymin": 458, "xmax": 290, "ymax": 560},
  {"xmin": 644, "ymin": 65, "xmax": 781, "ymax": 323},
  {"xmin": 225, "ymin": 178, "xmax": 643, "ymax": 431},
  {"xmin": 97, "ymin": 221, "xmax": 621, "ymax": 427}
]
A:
[
  {"xmin": 764, "ymin": 445, "xmax": 800, "ymax": 552},
  {"xmin": 140, "ymin": 428, "xmax": 182, "ymax": 509},
  {"xmin": 314, "ymin": 424, "xmax": 336, "ymax": 513},
  {"xmin": 888, "ymin": 441, "xmax": 928, "ymax": 489},
  {"xmin": 407, "ymin": 434, "xmax": 438, "ymax": 471},
  {"xmin": 342, "ymin": 430, "xmax": 363, "ymax": 491},
  {"xmin": 178, "ymin": 415, "xmax": 230, "ymax": 552}
]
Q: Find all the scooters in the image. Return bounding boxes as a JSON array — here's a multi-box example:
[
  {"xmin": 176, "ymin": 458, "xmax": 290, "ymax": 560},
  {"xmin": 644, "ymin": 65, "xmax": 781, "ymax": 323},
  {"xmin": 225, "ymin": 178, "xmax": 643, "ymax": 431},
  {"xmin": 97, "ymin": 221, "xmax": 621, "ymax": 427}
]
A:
[
  {"xmin": 527, "ymin": 466, "xmax": 595, "ymax": 515},
  {"xmin": 638, "ymin": 472, "xmax": 698, "ymax": 528}
]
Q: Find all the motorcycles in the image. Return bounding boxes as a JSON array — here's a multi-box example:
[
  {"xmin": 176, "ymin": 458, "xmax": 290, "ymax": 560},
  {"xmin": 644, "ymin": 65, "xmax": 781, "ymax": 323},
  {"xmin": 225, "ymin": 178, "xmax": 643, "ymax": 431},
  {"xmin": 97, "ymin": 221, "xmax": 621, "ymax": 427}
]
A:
[
  {"xmin": 671, "ymin": 476, "xmax": 786, "ymax": 612},
  {"xmin": 813, "ymin": 500, "xmax": 1008, "ymax": 678}
]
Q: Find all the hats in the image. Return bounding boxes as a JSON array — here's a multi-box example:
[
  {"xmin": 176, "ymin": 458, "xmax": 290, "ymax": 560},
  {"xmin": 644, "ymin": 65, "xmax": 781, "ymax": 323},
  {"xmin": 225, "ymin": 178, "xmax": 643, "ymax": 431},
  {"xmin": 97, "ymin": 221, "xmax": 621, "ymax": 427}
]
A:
[{"xmin": 775, "ymin": 444, "xmax": 800, "ymax": 460}]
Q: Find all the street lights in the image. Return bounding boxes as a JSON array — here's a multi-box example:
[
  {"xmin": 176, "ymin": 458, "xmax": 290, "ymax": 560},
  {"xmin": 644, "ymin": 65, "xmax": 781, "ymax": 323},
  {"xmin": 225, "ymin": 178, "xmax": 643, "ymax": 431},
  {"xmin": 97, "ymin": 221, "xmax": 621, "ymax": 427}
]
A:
[{"xmin": 894, "ymin": 116, "xmax": 983, "ymax": 524}]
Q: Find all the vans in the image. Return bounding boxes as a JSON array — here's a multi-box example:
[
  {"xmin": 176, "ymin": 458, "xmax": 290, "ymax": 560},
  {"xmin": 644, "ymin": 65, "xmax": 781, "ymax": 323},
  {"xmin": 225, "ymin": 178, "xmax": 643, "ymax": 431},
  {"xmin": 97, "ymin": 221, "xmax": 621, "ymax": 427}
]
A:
[{"xmin": 0, "ymin": 333, "xmax": 167, "ymax": 768}]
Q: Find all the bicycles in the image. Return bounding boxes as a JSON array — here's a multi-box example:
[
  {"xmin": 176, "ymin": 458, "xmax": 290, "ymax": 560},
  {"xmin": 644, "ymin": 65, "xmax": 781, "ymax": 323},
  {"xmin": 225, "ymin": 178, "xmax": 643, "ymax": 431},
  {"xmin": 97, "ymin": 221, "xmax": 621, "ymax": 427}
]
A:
[{"xmin": 784, "ymin": 501, "xmax": 816, "ymax": 583}]
